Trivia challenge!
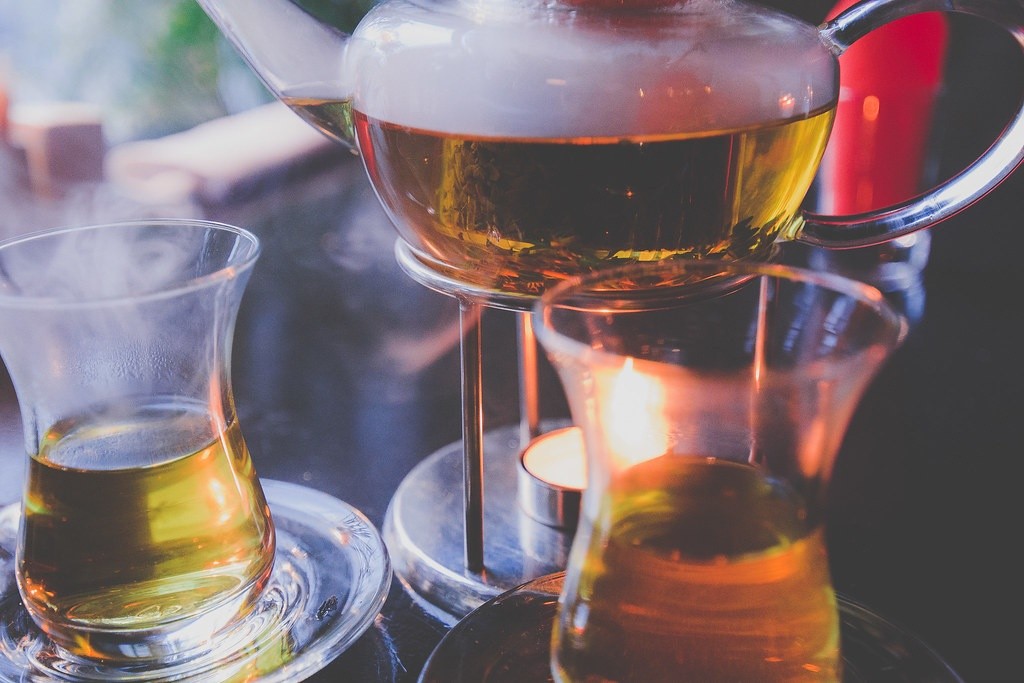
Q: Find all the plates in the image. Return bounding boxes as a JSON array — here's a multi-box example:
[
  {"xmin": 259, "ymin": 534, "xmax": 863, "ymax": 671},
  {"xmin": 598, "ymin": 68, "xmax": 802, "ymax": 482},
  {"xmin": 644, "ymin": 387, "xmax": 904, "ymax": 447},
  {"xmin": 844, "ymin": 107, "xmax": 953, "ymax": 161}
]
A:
[
  {"xmin": 0, "ymin": 477, "xmax": 392, "ymax": 682},
  {"xmin": 415, "ymin": 569, "xmax": 961, "ymax": 683}
]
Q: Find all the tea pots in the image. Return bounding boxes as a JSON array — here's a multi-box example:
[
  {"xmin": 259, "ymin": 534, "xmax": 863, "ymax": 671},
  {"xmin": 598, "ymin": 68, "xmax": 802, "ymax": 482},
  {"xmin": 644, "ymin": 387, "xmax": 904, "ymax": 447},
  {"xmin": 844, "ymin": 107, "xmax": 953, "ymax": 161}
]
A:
[{"xmin": 196, "ymin": 0, "xmax": 1024, "ymax": 277}]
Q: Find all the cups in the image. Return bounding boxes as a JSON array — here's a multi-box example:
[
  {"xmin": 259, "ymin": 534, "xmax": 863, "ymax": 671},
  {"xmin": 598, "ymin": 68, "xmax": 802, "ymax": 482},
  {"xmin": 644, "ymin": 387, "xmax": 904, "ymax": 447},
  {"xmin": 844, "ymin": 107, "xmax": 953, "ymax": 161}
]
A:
[
  {"xmin": 530, "ymin": 259, "xmax": 909, "ymax": 683},
  {"xmin": 0, "ymin": 217, "xmax": 275, "ymax": 661}
]
